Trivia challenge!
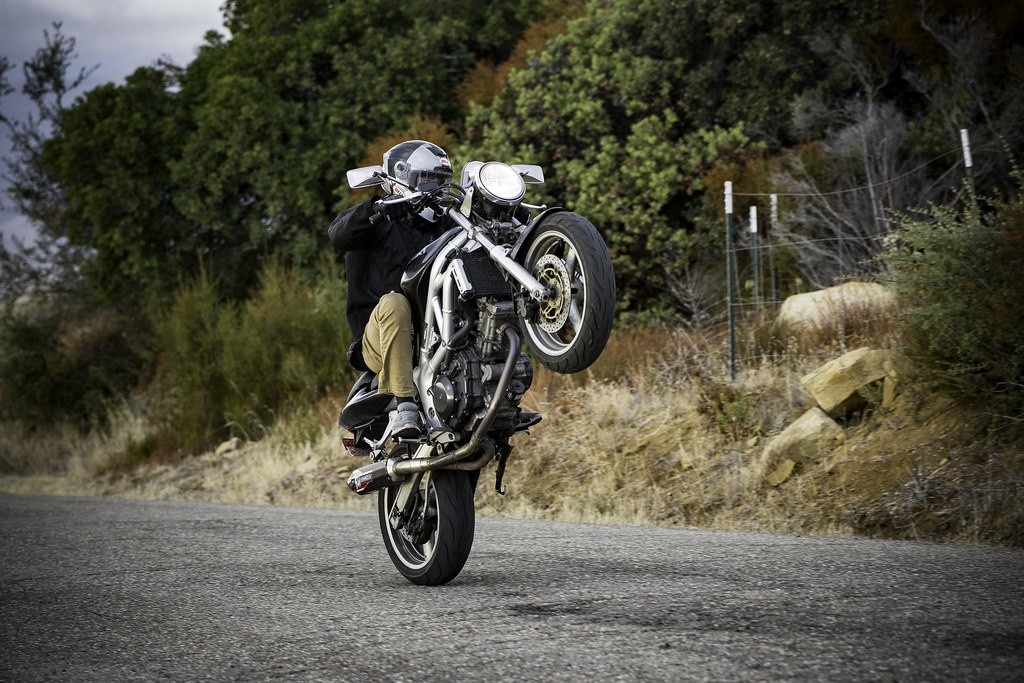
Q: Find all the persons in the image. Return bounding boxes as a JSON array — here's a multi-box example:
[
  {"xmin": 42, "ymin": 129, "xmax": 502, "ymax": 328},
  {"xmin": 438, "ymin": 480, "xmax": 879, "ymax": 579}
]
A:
[{"xmin": 328, "ymin": 140, "xmax": 531, "ymax": 443}]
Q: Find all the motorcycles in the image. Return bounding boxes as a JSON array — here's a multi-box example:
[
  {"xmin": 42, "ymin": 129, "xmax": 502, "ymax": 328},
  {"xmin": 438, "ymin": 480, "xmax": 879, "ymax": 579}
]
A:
[{"xmin": 339, "ymin": 160, "xmax": 616, "ymax": 585}]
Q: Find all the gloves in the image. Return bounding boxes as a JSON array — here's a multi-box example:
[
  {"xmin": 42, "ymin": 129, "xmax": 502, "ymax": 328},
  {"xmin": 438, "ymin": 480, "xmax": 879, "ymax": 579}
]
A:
[{"xmin": 372, "ymin": 193, "xmax": 415, "ymax": 223}]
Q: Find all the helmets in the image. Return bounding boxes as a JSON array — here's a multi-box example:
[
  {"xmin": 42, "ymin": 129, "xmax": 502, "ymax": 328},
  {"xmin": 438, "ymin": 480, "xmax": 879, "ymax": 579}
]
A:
[{"xmin": 380, "ymin": 139, "xmax": 453, "ymax": 225}]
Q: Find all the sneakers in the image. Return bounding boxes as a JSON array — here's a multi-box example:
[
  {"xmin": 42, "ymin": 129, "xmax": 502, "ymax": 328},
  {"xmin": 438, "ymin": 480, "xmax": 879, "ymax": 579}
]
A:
[{"xmin": 391, "ymin": 402, "xmax": 422, "ymax": 443}]
